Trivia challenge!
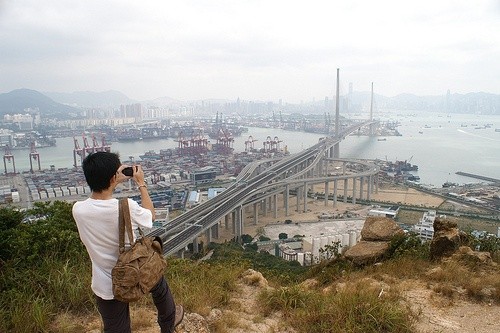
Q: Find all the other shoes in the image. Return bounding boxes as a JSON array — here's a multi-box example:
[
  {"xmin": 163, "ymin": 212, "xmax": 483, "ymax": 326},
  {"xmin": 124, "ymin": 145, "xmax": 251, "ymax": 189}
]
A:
[{"xmin": 162, "ymin": 304, "xmax": 184, "ymax": 332}]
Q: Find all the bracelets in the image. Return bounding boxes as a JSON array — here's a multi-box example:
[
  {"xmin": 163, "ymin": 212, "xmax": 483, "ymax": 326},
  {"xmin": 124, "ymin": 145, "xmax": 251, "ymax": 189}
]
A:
[{"xmin": 138, "ymin": 185, "xmax": 147, "ymax": 190}]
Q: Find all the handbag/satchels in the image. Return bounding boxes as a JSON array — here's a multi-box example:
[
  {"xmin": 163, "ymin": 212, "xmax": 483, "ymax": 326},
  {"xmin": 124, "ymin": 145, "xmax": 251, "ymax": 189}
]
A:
[{"xmin": 111, "ymin": 198, "xmax": 168, "ymax": 303}]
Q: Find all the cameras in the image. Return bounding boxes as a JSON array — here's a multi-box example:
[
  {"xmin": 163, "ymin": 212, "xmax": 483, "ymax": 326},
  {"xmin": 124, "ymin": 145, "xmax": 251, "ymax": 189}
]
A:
[{"xmin": 122, "ymin": 166, "xmax": 138, "ymax": 177}]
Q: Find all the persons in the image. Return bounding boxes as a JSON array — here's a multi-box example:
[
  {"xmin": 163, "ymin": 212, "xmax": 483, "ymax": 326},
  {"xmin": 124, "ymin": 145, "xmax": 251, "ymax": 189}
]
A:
[{"xmin": 72, "ymin": 152, "xmax": 184, "ymax": 333}]
